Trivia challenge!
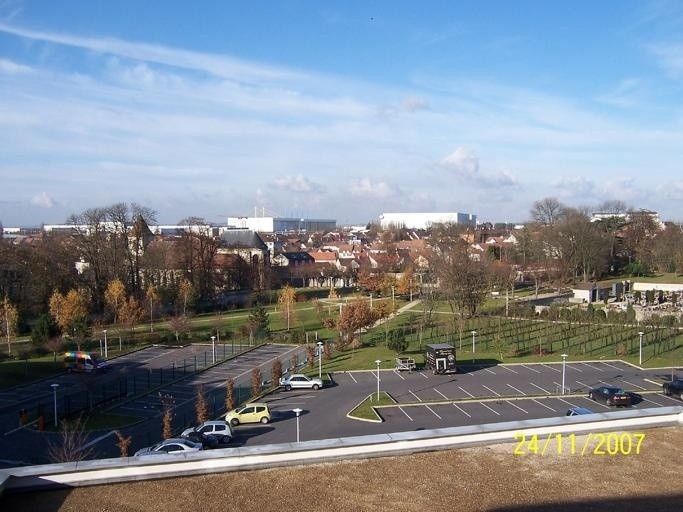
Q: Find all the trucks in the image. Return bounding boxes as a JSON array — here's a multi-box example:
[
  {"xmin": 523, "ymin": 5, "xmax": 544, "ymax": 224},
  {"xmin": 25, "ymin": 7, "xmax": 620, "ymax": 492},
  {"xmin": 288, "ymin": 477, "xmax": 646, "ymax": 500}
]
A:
[{"xmin": 422, "ymin": 343, "xmax": 457, "ymax": 375}]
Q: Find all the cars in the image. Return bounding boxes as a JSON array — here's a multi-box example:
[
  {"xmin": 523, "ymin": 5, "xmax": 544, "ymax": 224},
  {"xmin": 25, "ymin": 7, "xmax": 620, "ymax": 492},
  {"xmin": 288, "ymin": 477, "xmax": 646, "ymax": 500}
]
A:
[
  {"xmin": 225, "ymin": 402, "xmax": 273, "ymax": 426},
  {"xmin": 283, "ymin": 373, "xmax": 324, "ymax": 391},
  {"xmin": 133, "ymin": 420, "xmax": 234, "ymax": 457},
  {"xmin": 588, "ymin": 386, "xmax": 633, "ymax": 407},
  {"xmin": 663, "ymin": 379, "xmax": 683, "ymax": 402}
]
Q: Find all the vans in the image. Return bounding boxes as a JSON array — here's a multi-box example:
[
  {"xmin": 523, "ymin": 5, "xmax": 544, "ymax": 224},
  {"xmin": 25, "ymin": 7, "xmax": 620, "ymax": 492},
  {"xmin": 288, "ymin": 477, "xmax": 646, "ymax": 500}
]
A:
[
  {"xmin": 565, "ymin": 407, "xmax": 594, "ymax": 417},
  {"xmin": 63, "ymin": 350, "xmax": 109, "ymax": 375}
]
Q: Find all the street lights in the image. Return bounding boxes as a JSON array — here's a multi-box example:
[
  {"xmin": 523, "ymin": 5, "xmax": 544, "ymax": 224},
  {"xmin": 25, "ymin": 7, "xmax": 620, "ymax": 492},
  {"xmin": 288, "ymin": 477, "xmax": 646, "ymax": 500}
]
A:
[
  {"xmin": 316, "ymin": 341, "xmax": 323, "ymax": 379},
  {"xmin": 638, "ymin": 331, "xmax": 644, "ymax": 366},
  {"xmin": 470, "ymin": 330, "xmax": 477, "ymax": 354},
  {"xmin": 292, "ymin": 407, "xmax": 303, "ymax": 443},
  {"xmin": 50, "ymin": 383, "xmax": 59, "ymax": 428},
  {"xmin": 560, "ymin": 353, "xmax": 569, "ymax": 396},
  {"xmin": 102, "ymin": 329, "xmax": 108, "ymax": 358},
  {"xmin": 210, "ymin": 335, "xmax": 216, "ymax": 365},
  {"xmin": 374, "ymin": 359, "xmax": 382, "ymax": 401}
]
